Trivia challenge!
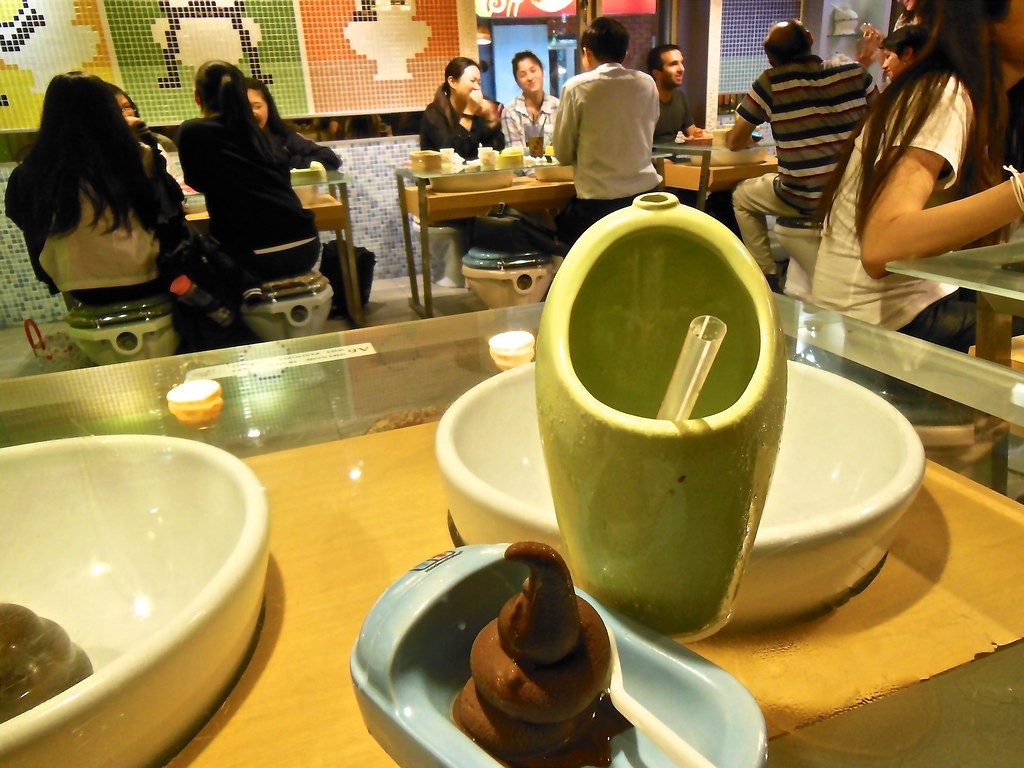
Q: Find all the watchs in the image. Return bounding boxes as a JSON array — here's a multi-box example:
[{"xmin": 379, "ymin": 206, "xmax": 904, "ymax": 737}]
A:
[{"xmin": 461, "ymin": 112, "xmax": 478, "ymax": 122}]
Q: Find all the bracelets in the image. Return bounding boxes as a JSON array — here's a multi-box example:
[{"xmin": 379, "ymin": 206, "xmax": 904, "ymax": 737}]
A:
[{"xmin": 1002, "ymin": 165, "xmax": 1024, "ymax": 212}]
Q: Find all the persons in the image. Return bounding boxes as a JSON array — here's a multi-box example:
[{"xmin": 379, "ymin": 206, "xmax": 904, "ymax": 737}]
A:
[
  {"xmin": 860, "ymin": 0, "xmax": 930, "ymax": 47},
  {"xmin": 501, "ymin": 49, "xmax": 560, "ymax": 247},
  {"xmin": 242, "ymin": 77, "xmax": 341, "ymax": 171},
  {"xmin": 725, "ymin": 18, "xmax": 880, "ymax": 294},
  {"xmin": 878, "ymin": 25, "xmax": 931, "ymax": 81},
  {"xmin": 813, "ymin": 0, "xmax": 1024, "ymax": 355},
  {"xmin": 419, "ymin": 58, "xmax": 506, "ymax": 245},
  {"xmin": 648, "ymin": 44, "xmax": 702, "ymax": 142},
  {"xmin": 103, "ymin": 81, "xmax": 175, "ymax": 151},
  {"xmin": 176, "ymin": 60, "xmax": 323, "ymax": 293},
  {"xmin": 552, "ymin": 17, "xmax": 663, "ymax": 245},
  {"xmin": 5, "ymin": 71, "xmax": 190, "ymax": 306}
]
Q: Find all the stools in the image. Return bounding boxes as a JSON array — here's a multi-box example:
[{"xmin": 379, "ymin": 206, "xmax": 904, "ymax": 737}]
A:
[
  {"xmin": 63, "ymin": 296, "xmax": 181, "ymax": 367},
  {"xmin": 413, "ymin": 221, "xmax": 473, "ymax": 287},
  {"xmin": 461, "ymin": 245, "xmax": 556, "ymax": 309},
  {"xmin": 767, "ymin": 215, "xmax": 824, "ymax": 283},
  {"xmin": 240, "ymin": 270, "xmax": 333, "ymax": 342}
]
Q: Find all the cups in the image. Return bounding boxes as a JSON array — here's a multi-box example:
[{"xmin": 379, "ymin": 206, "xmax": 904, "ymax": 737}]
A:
[
  {"xmin": 440, "ymin": 148, "xmax": 453, "ymax": 175},
  {"xmin": 478, "ymin": 147, "xmax": 524, "ymax": 171},
  {"xmin": 528, "ymin": 125, "xmax": 544, "ymax": 157},
  {"xmin": 487, "ymin": 331, "xmax": 535, "ymax": 371},
  {"xmin": 166, "ymin": 380, "xmax": 222, "ymax": 425}
]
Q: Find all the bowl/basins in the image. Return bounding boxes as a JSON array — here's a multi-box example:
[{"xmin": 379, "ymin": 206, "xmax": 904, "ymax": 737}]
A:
[
  {"xmin": 434, "ymin": 361, "xmax": 925, "ymax": 626},
  {"xmin": 0, "ymin": 435, "xmax": 268, "ymax": 767},
  {"xmin": 684, "ymin": 136, "xmax": 712, "ymax": 146}
]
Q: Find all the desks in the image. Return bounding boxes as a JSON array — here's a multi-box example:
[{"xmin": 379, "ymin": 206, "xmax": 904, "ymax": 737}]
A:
[{"xmin": 0, "ymin": 144, "xmax": 1023, "ymax": 768}]
